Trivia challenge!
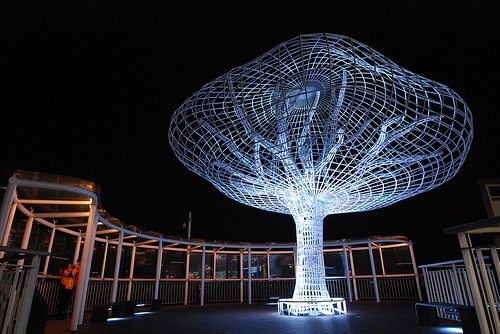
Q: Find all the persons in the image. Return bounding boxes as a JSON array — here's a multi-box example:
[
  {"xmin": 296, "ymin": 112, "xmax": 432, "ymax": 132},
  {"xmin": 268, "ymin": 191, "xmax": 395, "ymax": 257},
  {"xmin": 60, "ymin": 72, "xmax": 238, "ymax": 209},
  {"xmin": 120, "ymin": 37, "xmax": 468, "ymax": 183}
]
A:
[
  {"xmin": 56, "ymin": 262, "xmax": 76, "ymax": 319},
  {"xmin": 74, "ymin": 258, "xmax": 82, "ymax": 299}
]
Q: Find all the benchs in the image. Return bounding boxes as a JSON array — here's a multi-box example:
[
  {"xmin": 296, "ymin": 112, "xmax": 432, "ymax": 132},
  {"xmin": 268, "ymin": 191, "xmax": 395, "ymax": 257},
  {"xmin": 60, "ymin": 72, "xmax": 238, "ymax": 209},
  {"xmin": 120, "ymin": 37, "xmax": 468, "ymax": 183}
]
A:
[
  {"xmin": 416, "ymin": 300, "xmax": 480, "ymax": 334},
  {"xmin": 92, "ymin": 297, "xmax": 162, "ymax": 325}
]
